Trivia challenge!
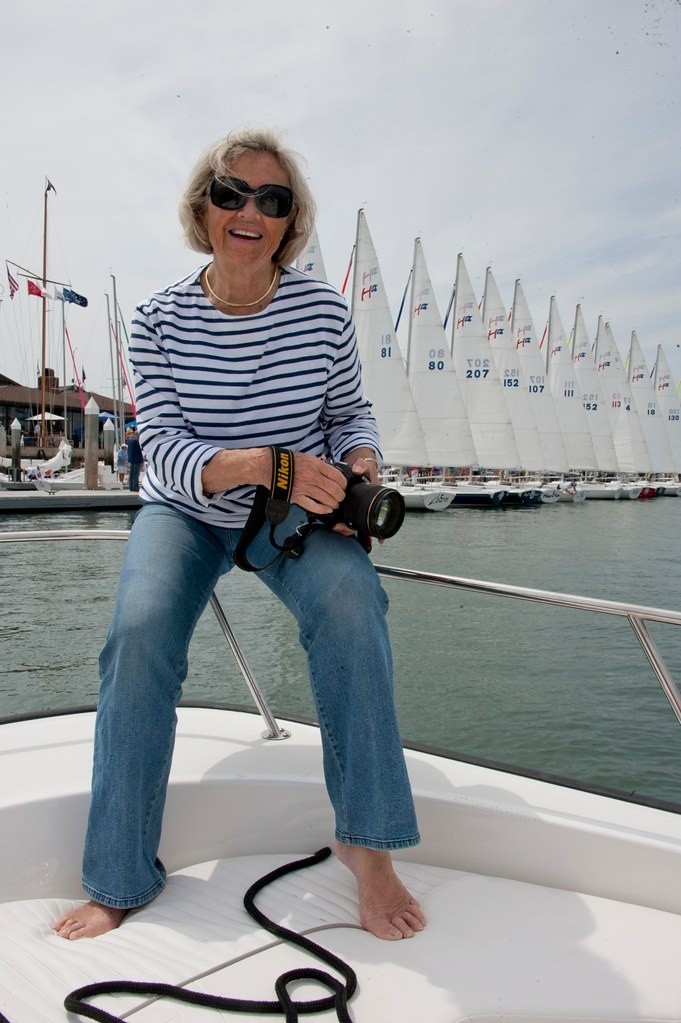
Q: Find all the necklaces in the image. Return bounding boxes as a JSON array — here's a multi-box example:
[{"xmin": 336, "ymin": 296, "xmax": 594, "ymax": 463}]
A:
[{"xmin": 206, "ymin": 262, "xmax": 278, "ymax": 307}]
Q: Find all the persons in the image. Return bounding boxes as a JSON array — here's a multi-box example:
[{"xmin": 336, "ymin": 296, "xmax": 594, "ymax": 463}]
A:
[
  {"xmin": 34, "ymin": 422, "xmax": 42, "ymax": 446},
  {"xmin": 117, "ymin": 431, "xmax": 144, "ymax": 492},
  {"xmin": 29, "ymin": 465, "xmax": 42, "ymax": 481},
  {"xmin": 52, "ymin": 132, "xmax": 427, "ymax": 942}
]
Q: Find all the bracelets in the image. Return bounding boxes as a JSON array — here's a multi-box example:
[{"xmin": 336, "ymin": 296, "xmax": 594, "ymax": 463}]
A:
[{"xmin": 357, "ymin": 458, "xmax": 379, "ymax": 469}]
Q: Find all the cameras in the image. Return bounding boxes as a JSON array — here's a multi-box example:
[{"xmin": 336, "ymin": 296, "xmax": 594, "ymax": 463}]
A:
[{"xmin": 308, "ymin": 460, "xmax": 405, "ymax": 535}]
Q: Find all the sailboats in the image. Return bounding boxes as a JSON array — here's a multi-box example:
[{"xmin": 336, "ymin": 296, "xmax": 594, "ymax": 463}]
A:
[
  {"xmin": 0, "ymin": 172, "xmax": 148, "ymax": 493},
  {"xmin": 295, "ymin": 199, "xmax": 681, "ymax": 512}
]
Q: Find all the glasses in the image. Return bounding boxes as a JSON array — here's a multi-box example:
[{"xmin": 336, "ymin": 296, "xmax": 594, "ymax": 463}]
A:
[{"xmin": 202, "ymin": 176, "xmax": 296, "ymax": 218}]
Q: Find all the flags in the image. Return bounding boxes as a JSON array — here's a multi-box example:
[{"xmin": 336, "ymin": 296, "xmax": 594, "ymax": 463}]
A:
[
  {"xmin": 6, "ymin": 263, "xmax": 19, "ymax": 299},
  {"xmin": 45, "ymin": 178, "xmax": 57, "ymax": 194},
  {"xmin": 27, "ymin": 280, "xmax": 88, "ymax": 308}
]
